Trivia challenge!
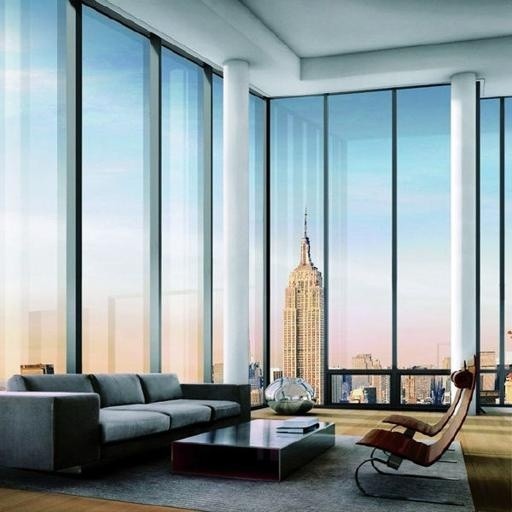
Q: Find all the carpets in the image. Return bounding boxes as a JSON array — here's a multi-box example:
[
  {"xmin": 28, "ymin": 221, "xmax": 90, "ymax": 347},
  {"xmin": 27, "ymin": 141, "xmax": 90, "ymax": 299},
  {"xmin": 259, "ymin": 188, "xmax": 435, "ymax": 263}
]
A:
[{"xmin": 0, "ymin": 433, "xmax": 478, "ymax": 512}]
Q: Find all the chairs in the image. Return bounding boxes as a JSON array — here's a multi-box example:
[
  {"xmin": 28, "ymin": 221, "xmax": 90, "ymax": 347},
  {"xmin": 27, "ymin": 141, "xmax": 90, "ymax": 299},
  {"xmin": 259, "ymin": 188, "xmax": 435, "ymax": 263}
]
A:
[{"xmin": 356, "ymin": 355, "xmax": 480, "ymax": 470}]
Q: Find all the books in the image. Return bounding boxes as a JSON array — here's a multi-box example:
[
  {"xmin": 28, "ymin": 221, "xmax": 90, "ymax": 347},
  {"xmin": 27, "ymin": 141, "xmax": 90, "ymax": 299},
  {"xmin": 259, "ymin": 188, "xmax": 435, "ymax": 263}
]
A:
[{"xmin": 276, "ymin": 421, "xmax": 320, "ymax": 434}]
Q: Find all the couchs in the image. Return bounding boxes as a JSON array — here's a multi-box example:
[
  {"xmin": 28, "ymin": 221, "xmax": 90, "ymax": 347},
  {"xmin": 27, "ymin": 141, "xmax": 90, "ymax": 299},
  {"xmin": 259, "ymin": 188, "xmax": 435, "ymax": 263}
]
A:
[{"xmin": 0, "ymin": 373, "xmax": 250, "ymax": 477}]
